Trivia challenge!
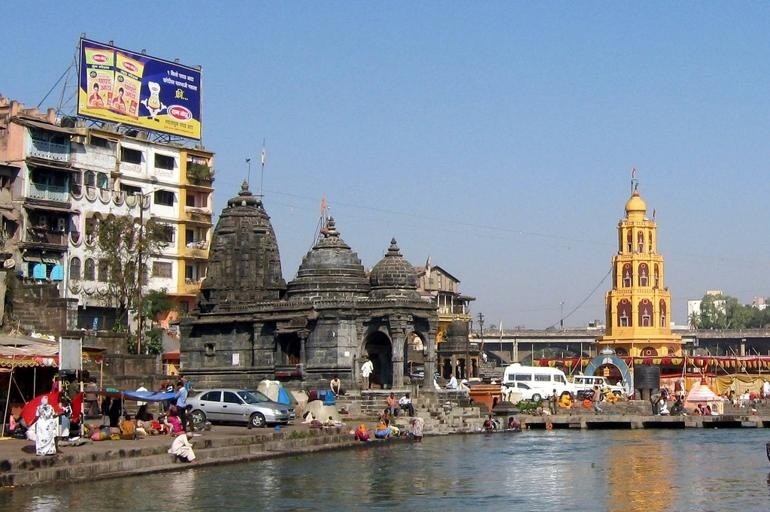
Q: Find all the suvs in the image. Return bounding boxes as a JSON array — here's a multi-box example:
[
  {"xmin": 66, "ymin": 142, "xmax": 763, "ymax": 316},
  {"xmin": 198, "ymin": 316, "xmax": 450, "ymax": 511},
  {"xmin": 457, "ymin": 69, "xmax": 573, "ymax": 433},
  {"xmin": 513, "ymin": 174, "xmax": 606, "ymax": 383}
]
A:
[{"xmin": 574, "ymin": 374, "xmax": 622, "ymax": 397}]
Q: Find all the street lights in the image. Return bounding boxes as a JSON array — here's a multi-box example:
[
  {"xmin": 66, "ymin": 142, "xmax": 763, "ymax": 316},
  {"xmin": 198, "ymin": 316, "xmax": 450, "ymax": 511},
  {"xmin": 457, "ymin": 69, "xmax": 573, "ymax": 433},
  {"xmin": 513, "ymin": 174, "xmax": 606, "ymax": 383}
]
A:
[{"xmin": 135, "ymin": 189, "xmax": 158, "ymax": 356}]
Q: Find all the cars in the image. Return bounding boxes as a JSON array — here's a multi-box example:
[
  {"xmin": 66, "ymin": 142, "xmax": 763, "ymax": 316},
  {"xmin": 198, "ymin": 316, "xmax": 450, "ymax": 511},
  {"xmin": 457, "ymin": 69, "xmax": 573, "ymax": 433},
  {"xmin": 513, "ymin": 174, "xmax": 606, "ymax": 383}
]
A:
[
  {"xmin": 505, "ymin": 381, "xmax": 549, "ymax": 402},
  {"xmin": 410, "ymin": 369, "xmax": 440, "ymax": 384},
  {"xmin": 186, "ymin": 388, "xmax": 296, "ymax": 427}
]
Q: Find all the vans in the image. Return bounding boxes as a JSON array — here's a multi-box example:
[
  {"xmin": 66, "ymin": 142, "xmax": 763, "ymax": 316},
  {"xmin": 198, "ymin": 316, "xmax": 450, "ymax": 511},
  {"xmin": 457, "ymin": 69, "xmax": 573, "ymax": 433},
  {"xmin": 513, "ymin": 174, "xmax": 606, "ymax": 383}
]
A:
[{"xmin": 503, "ymin": 362, "xmax": 576, "ymax": 397}]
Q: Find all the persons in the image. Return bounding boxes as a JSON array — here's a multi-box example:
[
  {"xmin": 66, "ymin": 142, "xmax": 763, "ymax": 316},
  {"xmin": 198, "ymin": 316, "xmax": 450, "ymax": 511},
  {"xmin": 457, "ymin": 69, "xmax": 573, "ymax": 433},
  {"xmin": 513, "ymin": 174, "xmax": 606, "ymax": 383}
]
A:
[
  {"xmin": 650, "ymin": 379, "xmax": 770, "ymax": 417},
  {"xmin": 113, "ymin": 88, "xmax": 126, "ymax": 111},
  {"xmin": 446, "ymin": 374, "xmax": 457, "ymax": 389},
  {"xmin": 311, "ymin": 358, "xmax": 424, "ymax": 442},
  {"xmin": 89, "ymin": 83, "xmax": 104, "ymax": 106},
  {"xmin": 550, "ymin": 386, "xmax": 615, "ymax": 415},
  {"xmin": 87, "ymin": 377, "xmax": 195, "ymax": 462},
  {"xmin": 480, "ymin": 414, "xmax": 520, "ymax": 432},
  {"xmin": 35, "ymin": 395, "xmax": 56, "ymax": 455}
]
